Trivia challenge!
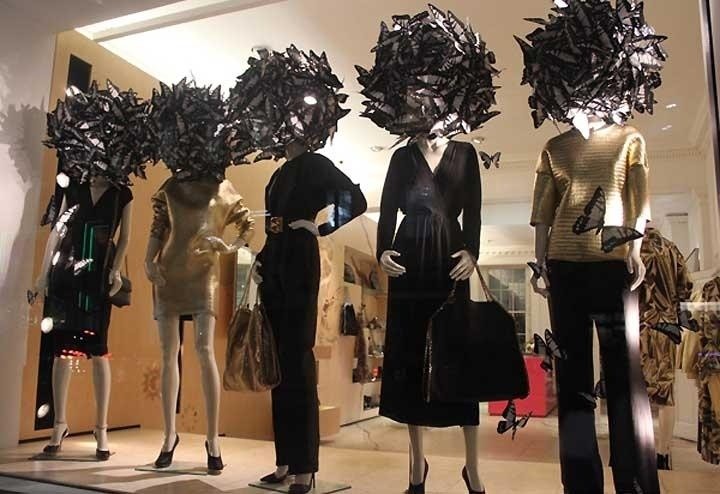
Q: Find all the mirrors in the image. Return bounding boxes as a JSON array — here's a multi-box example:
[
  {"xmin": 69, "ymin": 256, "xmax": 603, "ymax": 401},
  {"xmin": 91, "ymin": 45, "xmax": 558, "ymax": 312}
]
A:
[{"xmin": 233, "ymin": 245, "xmax": 261, "ymax": 311}]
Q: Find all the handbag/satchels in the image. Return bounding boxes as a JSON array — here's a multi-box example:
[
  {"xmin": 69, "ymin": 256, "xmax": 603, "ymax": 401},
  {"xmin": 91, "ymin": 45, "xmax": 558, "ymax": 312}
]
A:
[
  {"xmin": 420, "ymin": 249, "xmax": 530, "ymax": 404},
  {"xmin": 222, "ymin": 264, "xmax": 283, "ymax": 392},
  {"xmin": 102, "ymin": 266, "xmax": 131, "ymax": 308}
]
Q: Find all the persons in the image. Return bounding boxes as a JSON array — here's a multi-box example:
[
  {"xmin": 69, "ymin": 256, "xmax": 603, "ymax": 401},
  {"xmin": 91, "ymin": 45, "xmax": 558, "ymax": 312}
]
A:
[
  {"xmin": 641, "ymin": 223, "xmax": 689, "ymax": 471},
  {"xmin": 33, "ymin": 173, "xmax": 134, "ymax": 462},
  {"xmin": 694, "ymin": 275, "xmax": 719, "ymax": 464},
  {"xmin": 528, "ymin": 112, "xmax": 661, "ymax": 493},
  {"xmin": 249, "ymin": 144, "xmax": 369, "ymax": 494},
  {"xmin": 143, "ymin": 168, "xmax": 257, "ymax": 472},
  {"xmin": 374, "ymin": 124, "xmax": 487, "ymax": 493}
]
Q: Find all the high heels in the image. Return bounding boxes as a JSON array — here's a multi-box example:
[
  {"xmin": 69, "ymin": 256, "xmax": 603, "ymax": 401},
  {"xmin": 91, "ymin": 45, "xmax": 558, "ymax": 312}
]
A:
[
  {"xmin": 287, "ymin": 472, "xmax": 315, "ymax": 494},
  {"xmin": 260, "ymin": 470, "xmax": 289, "ymax": 483}
]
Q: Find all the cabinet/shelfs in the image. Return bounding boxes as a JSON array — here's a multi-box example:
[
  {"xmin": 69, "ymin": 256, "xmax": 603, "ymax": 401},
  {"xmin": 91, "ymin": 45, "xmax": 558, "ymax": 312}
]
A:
[{"xmin": 318, "ymin": 240, "xmax": 388, "ymax": 425}]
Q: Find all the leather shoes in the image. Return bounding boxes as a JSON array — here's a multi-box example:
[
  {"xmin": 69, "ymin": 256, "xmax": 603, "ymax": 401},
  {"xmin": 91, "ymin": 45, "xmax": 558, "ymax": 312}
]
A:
[
  {"xmin": 462, "ymin": 466, "xmax": 485, "ymax": 494},
  {"xmin": 657, "ymin": 452, "xmax": 669, "ymax": 470},
  {"xmin": 155, "ymin": 434, "xmax": 178, "ymax": 467},
  {"xmin": 43, "ymin": 429, "xmax": 67, "ymax": 452},
  {"xmin": 205, "ymin": 441, "xmax": 223, "ymax": 470},
  {"xmin": 93, "ymin": 430, "xmax": 109, "ymax": 459},
  {"xmin": 409, "ymin": 458, "xmax": 428, "ymax": 494}
]
